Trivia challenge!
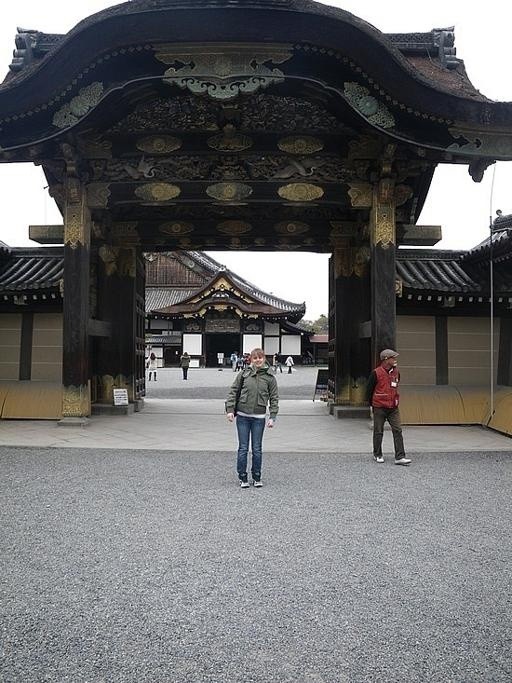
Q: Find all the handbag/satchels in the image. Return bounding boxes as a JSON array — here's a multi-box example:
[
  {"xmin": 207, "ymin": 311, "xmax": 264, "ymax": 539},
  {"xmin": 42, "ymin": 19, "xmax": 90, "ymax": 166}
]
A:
[{"xmin": 225, "ymin": 370, "xmax": 245, "ymax": 417}]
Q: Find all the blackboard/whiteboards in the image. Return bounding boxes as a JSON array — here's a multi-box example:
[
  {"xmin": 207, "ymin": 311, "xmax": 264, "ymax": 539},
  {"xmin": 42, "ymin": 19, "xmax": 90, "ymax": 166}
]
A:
[{"xmin": 315, "ymin": 369, "xmax": 329, "ymax": 395}]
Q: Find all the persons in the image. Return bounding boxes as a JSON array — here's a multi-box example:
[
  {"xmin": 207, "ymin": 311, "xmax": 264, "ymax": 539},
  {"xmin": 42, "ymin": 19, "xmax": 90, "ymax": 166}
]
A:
[
  {"xmin": 179, "ymin": 352, "xmax": 190, "ymax": 380},
  {"xmin": 226, "ymin": 348, "xmax": 279, "ymax": 488},
  {"xmin": 367, "ymin": 348, "xmax": 412, "ymax": 465},
  {"xmin": 231, "ymin": 350, "xmax": 295, "ymax": 374},
  {"xmin": 147, "ymin": 353, "xmax": 158, "ymax": 381}
]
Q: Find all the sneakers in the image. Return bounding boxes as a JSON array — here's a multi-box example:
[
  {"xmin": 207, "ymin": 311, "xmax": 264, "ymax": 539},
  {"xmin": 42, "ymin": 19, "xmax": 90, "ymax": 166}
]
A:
[
  {"xmin": 373, "ymin": 455, "xmax": 412, "ymax": 465},
  {"xmin": 240, "ymin": 478, "xmax": 263, "ymax": 488}
]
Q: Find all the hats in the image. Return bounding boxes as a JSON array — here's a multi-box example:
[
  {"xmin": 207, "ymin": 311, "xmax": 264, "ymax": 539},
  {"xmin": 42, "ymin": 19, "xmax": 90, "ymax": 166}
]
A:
[{"xmin": 380, "ymin": 349, "xmax": 400, "ymax": 361}]
